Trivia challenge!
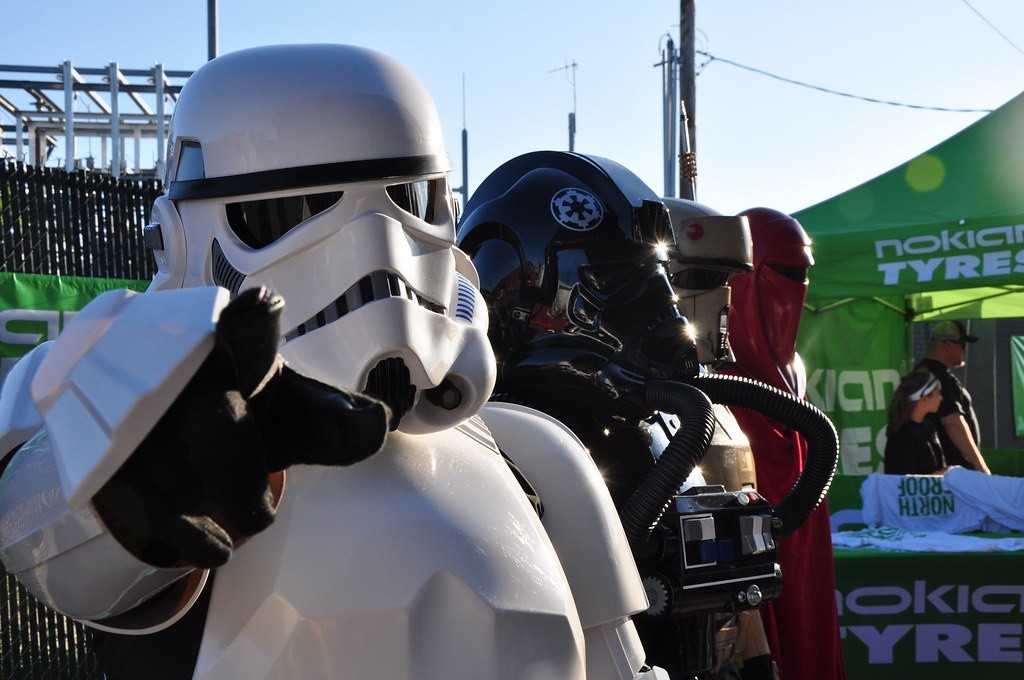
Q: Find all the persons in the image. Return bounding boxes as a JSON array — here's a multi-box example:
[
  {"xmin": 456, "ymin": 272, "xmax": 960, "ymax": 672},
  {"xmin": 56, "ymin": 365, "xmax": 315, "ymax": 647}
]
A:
[
  {"xmin": 0, "ymin": 42, "xmax": 847, "ymax": 680},
  {"xmin": 885, "ymin": 321, "xmax": 995, "ymax": 479}
]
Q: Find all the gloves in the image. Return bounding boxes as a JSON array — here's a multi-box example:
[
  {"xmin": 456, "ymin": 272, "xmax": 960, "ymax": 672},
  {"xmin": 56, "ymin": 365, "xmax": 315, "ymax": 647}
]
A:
[{"xmin": 93, "ymin": 286, "xmax": 394, "ymax": 569}]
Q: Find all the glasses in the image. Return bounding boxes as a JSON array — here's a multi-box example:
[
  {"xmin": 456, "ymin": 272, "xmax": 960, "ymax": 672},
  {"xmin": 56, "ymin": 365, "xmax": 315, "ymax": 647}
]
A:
[{"xmin": 942, "ymin": 338, "xmax": 967, "ymax": 348}]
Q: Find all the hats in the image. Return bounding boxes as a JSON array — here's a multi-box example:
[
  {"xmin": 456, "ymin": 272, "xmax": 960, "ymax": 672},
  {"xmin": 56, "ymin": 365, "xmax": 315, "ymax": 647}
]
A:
[{"xmin": 931, "ymin": 320, "xmax": 979, "ymax": 343}]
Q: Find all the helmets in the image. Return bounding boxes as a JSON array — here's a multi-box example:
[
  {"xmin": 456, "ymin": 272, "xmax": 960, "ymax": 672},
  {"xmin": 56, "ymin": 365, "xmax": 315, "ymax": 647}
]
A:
[
  {"xmin": 145, "ymin": 46, "xmax": 496, "ymax": 433},
  {"xmin": 730, "ymin": 206, "xmax": 814, "ymax": 357},
  {"xmin": 458, "ymin": 153, "xmax": 698, "ymax": 378},
  {"xmin": 658, "ymin": 200, "xmax": 753, "ymax": 361}
]
